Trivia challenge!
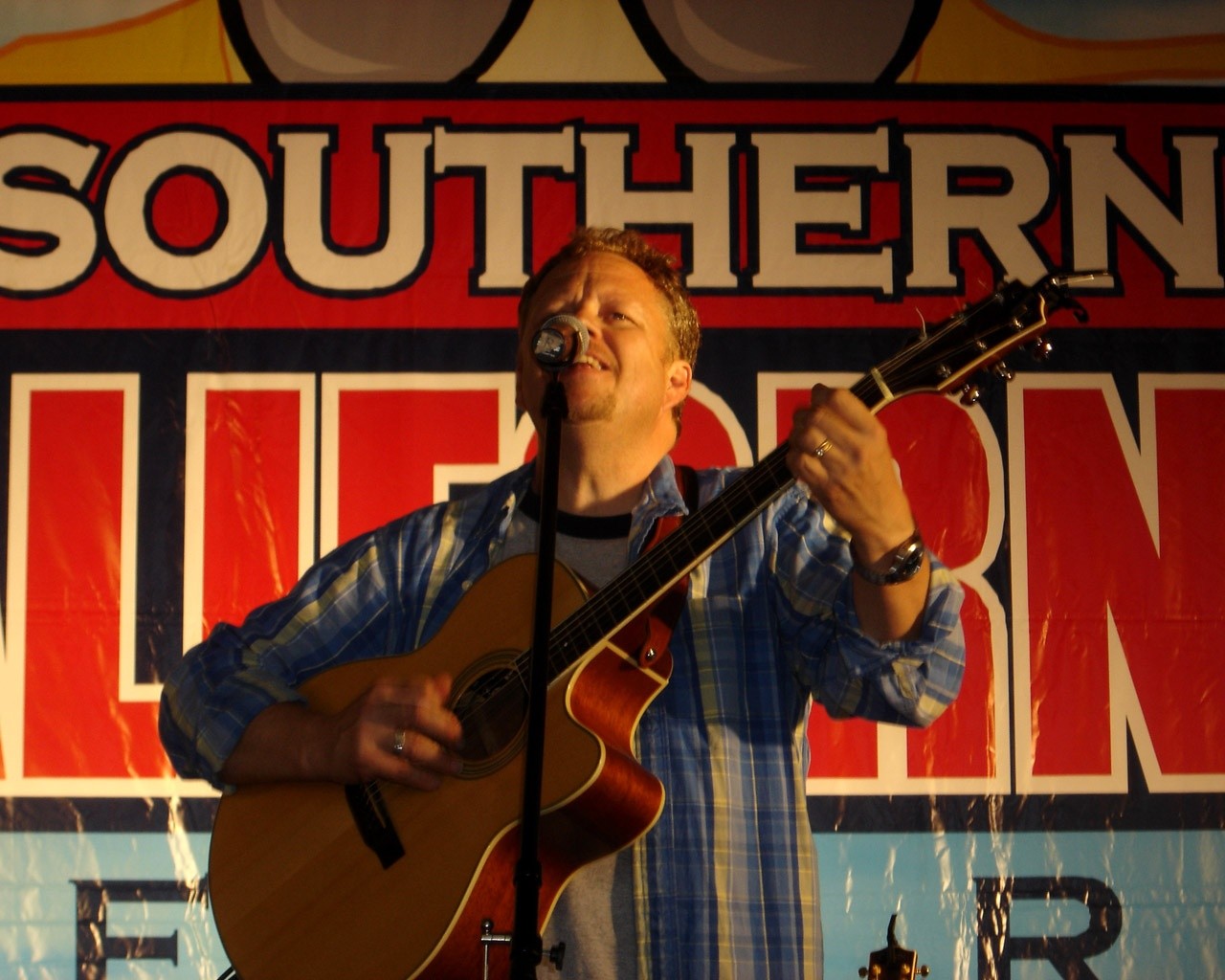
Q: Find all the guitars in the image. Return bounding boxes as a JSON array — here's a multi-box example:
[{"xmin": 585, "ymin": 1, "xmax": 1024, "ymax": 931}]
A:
[
  {"xmin": 209, "ymin": 266, "xmax": 1099, "ymax": 980},
  {"xmin": 858, "ymin": 910, "xmax": 928, "ymax": 980}
]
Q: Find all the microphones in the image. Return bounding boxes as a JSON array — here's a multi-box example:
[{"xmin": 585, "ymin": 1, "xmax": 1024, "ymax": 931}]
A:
[{"xmin": 534, "ymin": 311, "xmax": 591, "ymax": 371}]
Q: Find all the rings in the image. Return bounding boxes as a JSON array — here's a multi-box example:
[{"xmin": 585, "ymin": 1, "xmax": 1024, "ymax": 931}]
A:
[
  {"xmin": 391, "ymin": 726, "xmax": 405, "ymax": 752},
  {"xmin": 812, "ymin": 439, "xmax": 832, "ymax": 458}
]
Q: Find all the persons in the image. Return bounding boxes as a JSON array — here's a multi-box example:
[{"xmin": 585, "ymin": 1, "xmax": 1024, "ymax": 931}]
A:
[{"xmin": 155, "ymin": 224, "xmax": 971, "ymax": 980}]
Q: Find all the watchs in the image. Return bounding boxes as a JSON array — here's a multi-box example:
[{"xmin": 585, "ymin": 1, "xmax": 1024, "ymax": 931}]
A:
[{"xmin": 849, "ymin": 511, "xmax": 928, "ymax": 584}]
